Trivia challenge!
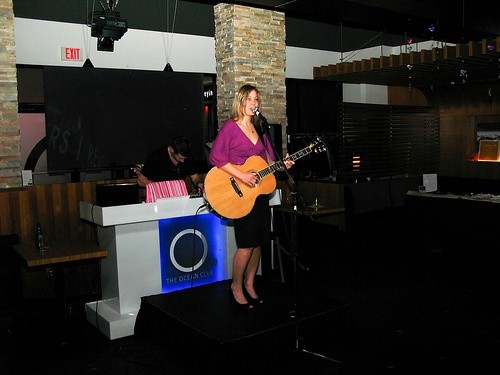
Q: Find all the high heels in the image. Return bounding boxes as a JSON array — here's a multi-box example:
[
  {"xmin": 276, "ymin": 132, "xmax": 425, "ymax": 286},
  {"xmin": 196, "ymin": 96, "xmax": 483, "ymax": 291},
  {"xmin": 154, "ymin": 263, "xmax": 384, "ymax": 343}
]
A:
[
  {"xmin": 229, "ymin": 286, "xmax": 254, "ymax": 310},
  {"xmin": 242, "ymin": 283, "xmax": 264, "ymax": 305}
]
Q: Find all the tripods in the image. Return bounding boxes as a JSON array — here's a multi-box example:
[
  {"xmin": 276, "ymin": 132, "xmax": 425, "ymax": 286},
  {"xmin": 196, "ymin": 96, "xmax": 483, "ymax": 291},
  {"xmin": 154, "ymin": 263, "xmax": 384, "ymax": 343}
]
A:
[{"xmin": 261, "ymin": 126, "xmax": 343, "ymax": 366}]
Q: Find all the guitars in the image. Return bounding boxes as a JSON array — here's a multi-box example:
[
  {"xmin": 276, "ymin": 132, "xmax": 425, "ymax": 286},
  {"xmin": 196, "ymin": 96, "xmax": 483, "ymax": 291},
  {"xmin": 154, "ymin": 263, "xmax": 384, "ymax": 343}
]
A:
[{"xmin": 203, "ymin": 136, "xmax": 327, "ymax": 222}]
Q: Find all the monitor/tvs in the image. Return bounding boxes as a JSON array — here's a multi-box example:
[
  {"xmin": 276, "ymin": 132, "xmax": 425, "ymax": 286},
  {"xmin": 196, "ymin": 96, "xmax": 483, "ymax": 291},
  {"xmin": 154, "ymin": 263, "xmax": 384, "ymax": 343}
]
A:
[{"xmin": 96, "ymin": 182, "xmax": 140, "ymax": 207}]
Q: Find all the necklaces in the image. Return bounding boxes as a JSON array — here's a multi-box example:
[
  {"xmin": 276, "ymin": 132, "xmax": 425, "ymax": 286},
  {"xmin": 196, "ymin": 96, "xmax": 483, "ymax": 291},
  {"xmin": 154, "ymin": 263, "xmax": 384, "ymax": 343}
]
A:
[{"xmin": 242, "ymin": 121, "xmax": 255, "ymax": 134}]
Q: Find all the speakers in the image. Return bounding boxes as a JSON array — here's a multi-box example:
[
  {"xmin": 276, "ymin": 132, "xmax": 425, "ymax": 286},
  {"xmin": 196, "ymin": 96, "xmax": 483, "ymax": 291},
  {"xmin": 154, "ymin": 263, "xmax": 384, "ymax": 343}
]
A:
[{"xmin": 286, "ymin": 78, "xmax": 340, "ymax": 136}]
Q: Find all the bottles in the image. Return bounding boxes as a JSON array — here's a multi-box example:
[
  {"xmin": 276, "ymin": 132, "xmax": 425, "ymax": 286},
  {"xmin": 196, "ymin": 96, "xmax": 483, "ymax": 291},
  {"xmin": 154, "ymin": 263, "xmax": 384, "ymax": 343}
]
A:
[
  {"xmin": 28, "ymin": 179, "xmax": 33, "ymax": 186},
  {"xmin": 293, "ymin": 197, "xmax": 298, "ymax": 212},
  {"xmin": 35, "ymin": 224, "xmax": 44, "ymax": 251}
]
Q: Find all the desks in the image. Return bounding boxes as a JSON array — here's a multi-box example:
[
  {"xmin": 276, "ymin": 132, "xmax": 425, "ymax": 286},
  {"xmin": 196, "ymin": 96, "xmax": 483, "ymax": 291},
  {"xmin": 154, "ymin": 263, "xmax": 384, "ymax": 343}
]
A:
[
  {"xmin": 271, "ymin": 201, "xmax": 347, "ymax": 262},
  {"xmin": 407, "ymin": 188, "xmax": 499, "ymax": 247}
]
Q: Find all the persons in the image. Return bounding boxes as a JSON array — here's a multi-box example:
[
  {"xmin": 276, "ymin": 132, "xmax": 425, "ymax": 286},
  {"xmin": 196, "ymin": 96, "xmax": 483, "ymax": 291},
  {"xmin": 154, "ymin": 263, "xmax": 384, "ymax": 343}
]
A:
[
  {"xmin": 137, "ymin": 137, "xmax": 204, "ymax": 196},
  {"xmin": 208, "ymin": 84, "xmax": 296, "ymax": 309}
]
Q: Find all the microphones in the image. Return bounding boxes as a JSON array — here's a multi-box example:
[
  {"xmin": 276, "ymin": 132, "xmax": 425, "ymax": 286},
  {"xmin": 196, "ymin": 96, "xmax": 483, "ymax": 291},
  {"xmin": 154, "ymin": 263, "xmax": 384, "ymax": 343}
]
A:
[{"xmin": 253, "ymin": 108, "xmax": 270, "ymax": 129}]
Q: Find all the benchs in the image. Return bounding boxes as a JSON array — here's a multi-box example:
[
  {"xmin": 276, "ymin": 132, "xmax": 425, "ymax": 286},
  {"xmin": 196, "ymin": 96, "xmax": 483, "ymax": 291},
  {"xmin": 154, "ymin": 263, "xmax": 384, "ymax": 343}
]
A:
[
  {"xmin": 11, "ymin": 175, "xmax": 153, "ymax": 301},
  {"xmin": 353, "ymin": 177, "xmax": 431, "ymax": 223},
  {"xmin": 276, "ymin": 179, "xmax": 349, "ymax": 230}
]
